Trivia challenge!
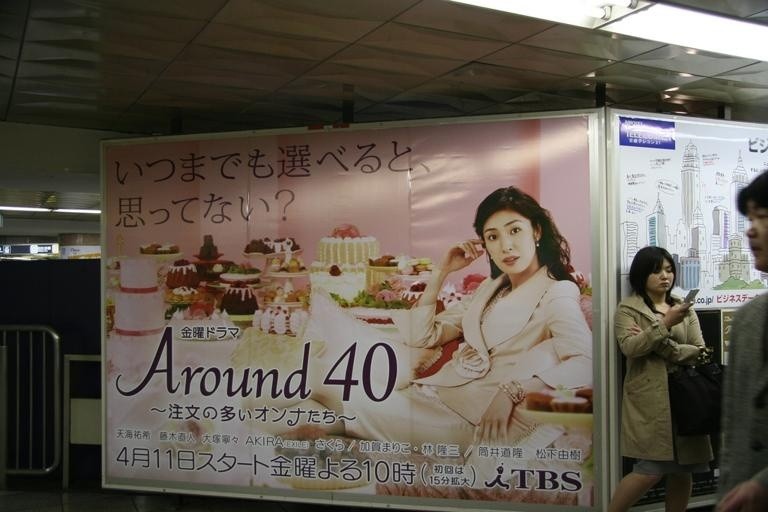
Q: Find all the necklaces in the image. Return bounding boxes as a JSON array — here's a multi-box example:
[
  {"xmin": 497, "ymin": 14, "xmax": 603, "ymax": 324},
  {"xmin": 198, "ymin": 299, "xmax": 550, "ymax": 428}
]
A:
[{"xmin": 478, "ymin": 284, "xmax": 511, "ymax": 325}]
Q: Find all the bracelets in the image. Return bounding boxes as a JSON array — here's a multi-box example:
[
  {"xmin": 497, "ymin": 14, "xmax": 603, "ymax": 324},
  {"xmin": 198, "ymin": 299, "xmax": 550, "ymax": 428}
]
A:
[
  {"xmin": 511, "ymin": 379, "xmax": 527, "ymax": 399},
  {"xmin": 497, "ymin": 384, "xmax": 520, "ymax": 407}
]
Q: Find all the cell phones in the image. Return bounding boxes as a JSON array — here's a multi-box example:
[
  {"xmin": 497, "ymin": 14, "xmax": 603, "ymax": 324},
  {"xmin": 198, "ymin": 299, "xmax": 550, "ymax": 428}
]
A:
[{"xmin": 683, "ymin": 288, "xmax": 700, "ymax": 303}]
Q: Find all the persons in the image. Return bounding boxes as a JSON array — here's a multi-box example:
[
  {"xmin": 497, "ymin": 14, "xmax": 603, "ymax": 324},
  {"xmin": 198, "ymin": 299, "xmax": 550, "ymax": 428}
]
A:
[
  {"xmin": 607, "ymin": 245, "xmax": 714, "ymax": 512},
  {"xmin": 240, "ymin": 186, "xmax": 595, "ymax": 451},
  {"xmin": 715, "ymin": 171, "xmax": 768, "ymax": 511}
]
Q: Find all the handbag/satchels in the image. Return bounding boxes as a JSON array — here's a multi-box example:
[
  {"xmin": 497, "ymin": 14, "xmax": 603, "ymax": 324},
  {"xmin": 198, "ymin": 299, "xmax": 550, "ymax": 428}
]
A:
[{"xmin": 668, "ymin": 362, "xmax": 724, "ymax": 434}]
